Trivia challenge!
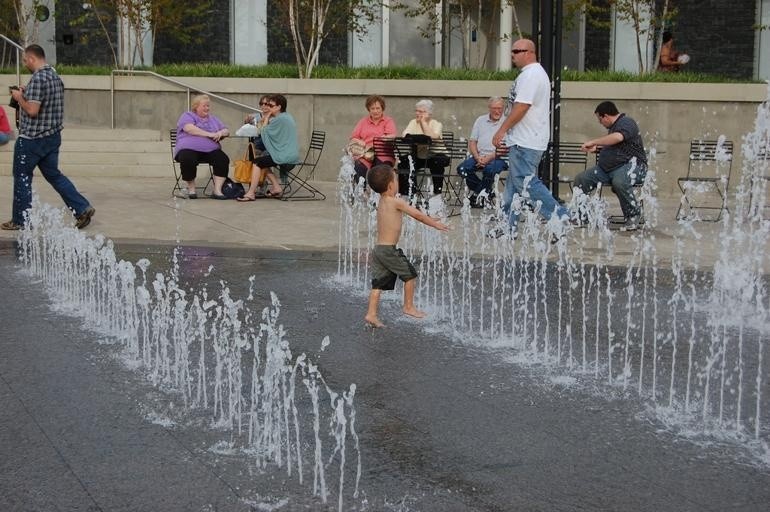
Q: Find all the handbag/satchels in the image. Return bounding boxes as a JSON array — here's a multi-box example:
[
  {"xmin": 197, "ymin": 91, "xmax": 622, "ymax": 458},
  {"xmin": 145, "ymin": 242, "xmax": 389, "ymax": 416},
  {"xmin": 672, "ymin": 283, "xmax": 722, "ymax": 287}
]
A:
[
  {"xmin": 348, "ymin": 138, "xmax": 374, "ymax": 162},
  {"xmin": 235, "ymin": 123, "xmax": 259, "ymax": 136},
  {"xmin": 232, "ymin": 160, "xmax": 265, "ymax": 185},
  {"xmin": 222, "ymin": 177, "xmax": 245, "ymax": 199}
]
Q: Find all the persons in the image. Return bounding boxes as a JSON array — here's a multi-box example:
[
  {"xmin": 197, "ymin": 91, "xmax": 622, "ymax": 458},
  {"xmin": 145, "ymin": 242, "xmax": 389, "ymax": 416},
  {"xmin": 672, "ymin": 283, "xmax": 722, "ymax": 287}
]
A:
[
  {"xmin": 573, "ymin": 101, "xmax": 648, "ymax": 231},
  {"xmin": 0, "ymin": 108, "xmax": 11, "ymax": 145},
  {"xmin": 0, "ymin": 45, "xmax": 96, "ymax": 231},
  {"xmin": 484, "ymin": 37, "xmax": 572, "ymax": 245},
  {"xmin": 397, "ymin": 98, "xmax": 450, "ymax": 196},
  {"xmin": 172, "ymin": 92, "xmax": 301, "ymax": 202},
  {"xmin": 363, "ymin": 163, "xmax": 450, "ymax": 328},
  {"xmin": 348, "ymin": 96, "xmax": 396, "ymax": 209},
  {"xmin": 456, "ymin": 96, "xmax": 508, "ymax": 209},
  {"xmin": 657, "ymin": 32, "xmax": 683, "ymax": 72}
]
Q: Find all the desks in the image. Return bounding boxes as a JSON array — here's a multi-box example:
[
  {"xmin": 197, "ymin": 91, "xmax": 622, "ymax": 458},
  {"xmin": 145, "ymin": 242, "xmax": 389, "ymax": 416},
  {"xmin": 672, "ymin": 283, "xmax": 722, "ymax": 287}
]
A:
[{"xmin": 222, "ymin": 135, "xmax": 267, "ymax": 198}]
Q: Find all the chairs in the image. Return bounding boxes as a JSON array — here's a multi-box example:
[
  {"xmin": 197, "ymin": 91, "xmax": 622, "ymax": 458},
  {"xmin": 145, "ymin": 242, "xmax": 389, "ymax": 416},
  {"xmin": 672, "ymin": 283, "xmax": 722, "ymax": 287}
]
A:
[
  {"xmin": 676, "ymin": 140, "xmax": 733, "ymax": 222},
  {"xmin": 747, "ymin": 136, "xmax": 770, "ymax": 220},
  {"xmin": 170, "ymin": 129, "xmax": 215, "ymax": 199},
  {"xmin": 348, "ymin": 132, "xmax": 645, "ymax": 232},
  {"xmin": 274, "ymin": 131, "xmax": 326, "ymax": 201}
]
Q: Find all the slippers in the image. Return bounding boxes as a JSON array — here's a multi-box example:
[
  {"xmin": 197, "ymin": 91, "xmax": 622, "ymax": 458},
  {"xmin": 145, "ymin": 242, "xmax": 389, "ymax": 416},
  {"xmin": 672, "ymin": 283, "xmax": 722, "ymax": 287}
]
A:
[
  {"xmin": 265, "ymin": 190, "xmax": 284, "ymax": 198},
  {"xmin": 236, "ymin": 195, "xmax": 255, "ymax": 201}
]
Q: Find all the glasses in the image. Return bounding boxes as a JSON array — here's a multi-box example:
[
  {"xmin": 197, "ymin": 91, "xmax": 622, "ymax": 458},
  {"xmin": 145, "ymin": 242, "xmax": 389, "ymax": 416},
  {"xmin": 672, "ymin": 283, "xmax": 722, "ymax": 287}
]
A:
[
  {"xmin": 260, "ymin": 102, "xmax": 268, "ymax": 106},
  {"xmin": 268, "ymin": 104, "xmax": 276, "ymax": 108},
  {"xmin": 511, "ymin": 49, "xmax": 528, "ymax": 54}
]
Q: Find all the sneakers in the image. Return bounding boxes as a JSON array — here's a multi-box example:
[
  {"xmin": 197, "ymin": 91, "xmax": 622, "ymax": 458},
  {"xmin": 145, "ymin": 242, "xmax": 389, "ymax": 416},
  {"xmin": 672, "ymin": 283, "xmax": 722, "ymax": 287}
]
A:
[
  {"xmin": 74, "ymin": 206, "xmax": 95, "ymax": 229},
  {"xmin": 1, "ymin": 221, "xmax": 33, "ymax": 230},
  {"xmin": 625, "ymin": 215, "xmax": 640, "ymax": 231},
  {"xmin": 486, "ymin": 227, "xmax": 518, "ymax": 239}
]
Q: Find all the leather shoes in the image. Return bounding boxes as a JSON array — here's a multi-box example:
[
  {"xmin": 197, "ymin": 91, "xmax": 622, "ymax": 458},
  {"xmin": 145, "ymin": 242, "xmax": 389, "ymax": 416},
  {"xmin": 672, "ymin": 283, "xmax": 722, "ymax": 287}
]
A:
[
  {"xmin": 213, "ymin": 194, "xmax": 226, "ymax": 200},
  {"xmin": 189, "ymin": 194, "xmax": 196, "ymax": 199}
]
